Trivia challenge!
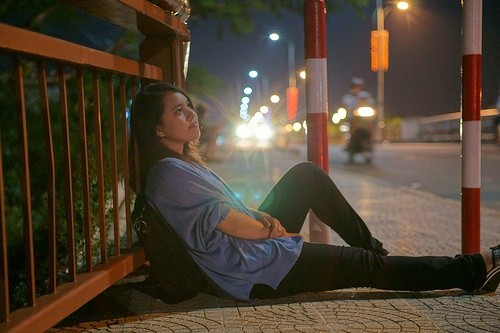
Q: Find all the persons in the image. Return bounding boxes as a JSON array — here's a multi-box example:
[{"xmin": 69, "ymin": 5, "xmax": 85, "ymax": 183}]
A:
[
  {"xmin": 340, "ymin": 77, "xmax": 376, "ymax": 152},
  {"xmin": 130, "ymin": 83, "xmax": 500, "ymax": 302}
]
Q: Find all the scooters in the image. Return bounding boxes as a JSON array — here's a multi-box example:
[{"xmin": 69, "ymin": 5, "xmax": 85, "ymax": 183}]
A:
[{"xmin": 342, "ymin": 107, "xmax": 376, "ymax": 166}]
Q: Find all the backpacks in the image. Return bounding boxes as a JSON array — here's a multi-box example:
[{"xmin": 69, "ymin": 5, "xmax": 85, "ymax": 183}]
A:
[{"xmin": 131, "ymin": 196, "xmax": 204, "ymax": 304}]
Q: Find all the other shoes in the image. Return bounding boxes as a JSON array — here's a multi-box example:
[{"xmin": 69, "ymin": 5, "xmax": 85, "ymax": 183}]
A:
[{"xmin": 481, "ymin": 244, "xmax": 500, "ymax": 292}]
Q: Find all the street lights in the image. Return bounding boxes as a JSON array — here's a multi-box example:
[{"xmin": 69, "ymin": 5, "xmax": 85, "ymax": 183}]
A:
[{"xmin": 374, "ymin": 1, "xmax": 411, "ymax": 129}]
